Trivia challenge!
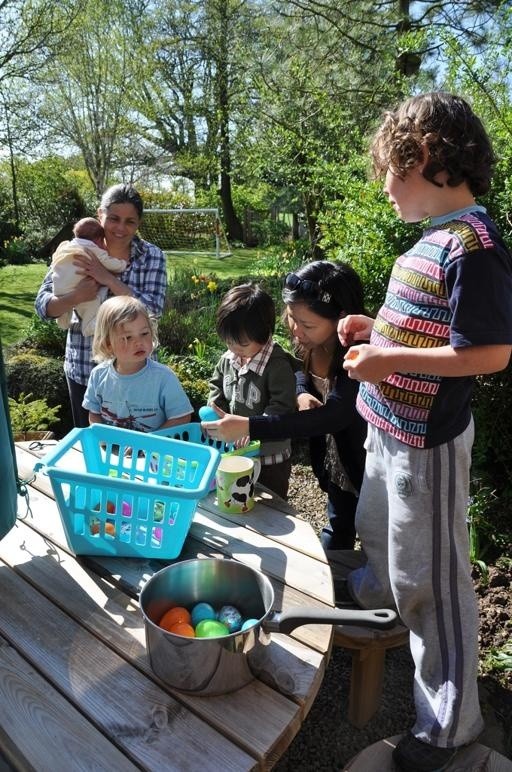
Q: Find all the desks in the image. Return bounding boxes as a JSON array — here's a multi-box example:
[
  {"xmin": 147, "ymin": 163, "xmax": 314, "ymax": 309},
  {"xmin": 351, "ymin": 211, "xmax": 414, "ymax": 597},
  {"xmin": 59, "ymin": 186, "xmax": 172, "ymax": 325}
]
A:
[{"xmin": 0, "ymin": 441, "xmax": 336, "ymax": 772}]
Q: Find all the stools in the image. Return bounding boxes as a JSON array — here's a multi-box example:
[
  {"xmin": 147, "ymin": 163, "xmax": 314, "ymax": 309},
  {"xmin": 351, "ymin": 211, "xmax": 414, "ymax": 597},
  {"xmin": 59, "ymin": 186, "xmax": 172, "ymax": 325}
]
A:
[
  {"xmin": 340, "ymin": 732, "xmax": 511, "ymax": 772},
  {"xmin": 325, "ymin": 549, "xmax": 409, "ymax": 730}
]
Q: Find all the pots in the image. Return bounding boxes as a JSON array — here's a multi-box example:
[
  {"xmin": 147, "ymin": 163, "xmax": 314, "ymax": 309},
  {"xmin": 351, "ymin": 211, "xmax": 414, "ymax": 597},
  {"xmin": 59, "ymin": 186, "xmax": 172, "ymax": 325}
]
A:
[{"xmin": 142, "ymin": 557, "xmax": 396, "ymax": 698}]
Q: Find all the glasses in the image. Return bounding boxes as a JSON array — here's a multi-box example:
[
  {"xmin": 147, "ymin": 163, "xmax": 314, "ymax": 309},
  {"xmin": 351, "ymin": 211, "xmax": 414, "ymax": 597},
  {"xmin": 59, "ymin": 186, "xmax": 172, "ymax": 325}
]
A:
[{"xmin": 283, "ymin": 271, "xmax": 335, "ymax": 306}]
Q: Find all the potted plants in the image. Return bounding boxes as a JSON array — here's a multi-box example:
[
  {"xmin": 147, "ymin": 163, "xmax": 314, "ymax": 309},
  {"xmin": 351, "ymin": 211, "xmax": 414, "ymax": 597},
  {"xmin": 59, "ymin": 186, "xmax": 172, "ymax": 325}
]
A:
[{"xmin": 8, "ymin": 391, "xmax": 62, "ymax": 441}]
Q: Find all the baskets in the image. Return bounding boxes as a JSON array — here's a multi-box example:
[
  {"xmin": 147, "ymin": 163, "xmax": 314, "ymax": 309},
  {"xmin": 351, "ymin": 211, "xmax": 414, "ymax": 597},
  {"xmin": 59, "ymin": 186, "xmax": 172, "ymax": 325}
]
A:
[
  {"xmin": 142, "ymin": 421, "xmax": 262, "ymax": 494},
  {"xmin": 32, "ymin": 423, "xmax": 221, "ymax": 559}
]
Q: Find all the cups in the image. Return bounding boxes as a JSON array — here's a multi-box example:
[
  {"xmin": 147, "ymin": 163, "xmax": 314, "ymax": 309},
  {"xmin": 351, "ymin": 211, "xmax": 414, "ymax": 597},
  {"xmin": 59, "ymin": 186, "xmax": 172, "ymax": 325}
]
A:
[{"xmin": 214, "ymin": 454, "xmax": 263, "ymax": 516}]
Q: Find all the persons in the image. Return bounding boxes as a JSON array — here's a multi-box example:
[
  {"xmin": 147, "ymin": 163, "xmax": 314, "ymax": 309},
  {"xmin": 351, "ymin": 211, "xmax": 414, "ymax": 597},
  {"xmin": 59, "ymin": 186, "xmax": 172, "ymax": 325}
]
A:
[
  {"xmin": 35, "ymin": 183, "xmax": 168, "ymax": 427},
  {"xmin": 335, "ymin": 93, "xmax": 509, "ymax": 770},
  {"xmin": 52, "ymin": 218, "xmax": 127, "ymax": 335},
  {"xmin": 80, "ymin": 296, "xmax": 194, "ymax": 457},
  {"xmin": 205, "ymin": 281, "xmax": 296, "ymax": 501},
  {"xmin": 199, "ymin": 261, "xmax": 376, "ymax": 548}
]
